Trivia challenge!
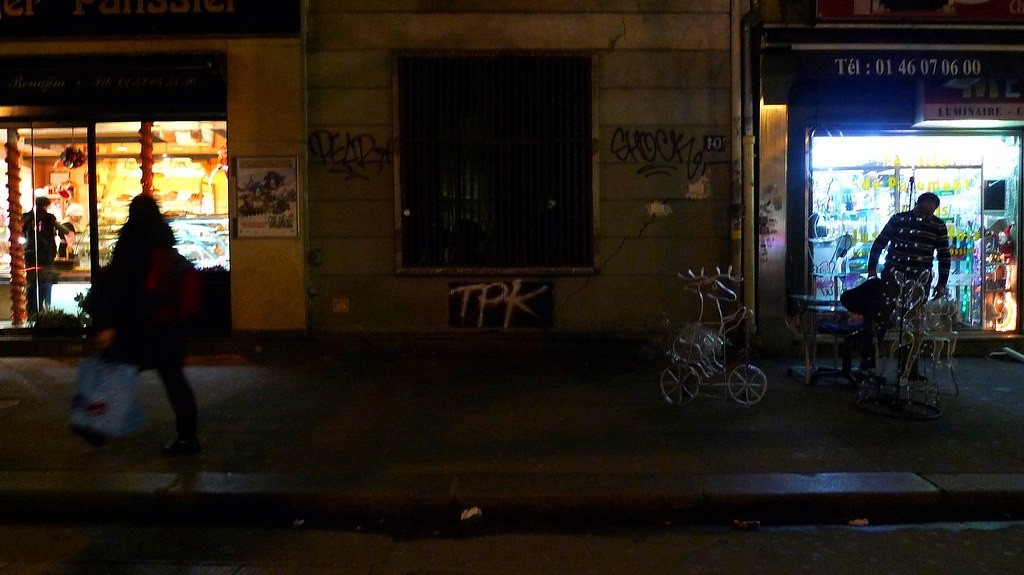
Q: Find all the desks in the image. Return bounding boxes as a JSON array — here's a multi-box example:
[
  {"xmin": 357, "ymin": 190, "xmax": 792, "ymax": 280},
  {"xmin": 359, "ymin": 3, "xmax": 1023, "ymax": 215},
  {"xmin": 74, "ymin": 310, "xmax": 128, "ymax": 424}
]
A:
[{"xmin": 788, "ymin": 293, "xmax": 862, "ymax": 386}]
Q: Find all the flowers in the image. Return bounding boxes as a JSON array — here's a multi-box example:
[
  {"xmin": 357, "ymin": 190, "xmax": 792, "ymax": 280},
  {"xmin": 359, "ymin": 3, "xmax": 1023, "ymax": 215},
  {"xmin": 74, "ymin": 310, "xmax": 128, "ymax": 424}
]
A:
[{"xmin": 59, "ymin": 146, "xmax": 86, "ymax": 170}]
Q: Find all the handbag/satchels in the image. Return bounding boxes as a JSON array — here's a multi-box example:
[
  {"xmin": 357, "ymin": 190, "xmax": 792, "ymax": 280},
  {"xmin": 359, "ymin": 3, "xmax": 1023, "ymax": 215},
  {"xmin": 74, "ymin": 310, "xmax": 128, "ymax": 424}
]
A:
[
  {"xmin": 68, "ymin": 359, "xmax": 144, "ymax": 436},
  {"xmin": 841, "ymin": 273, "xmax": 896, "ymax": 316}
]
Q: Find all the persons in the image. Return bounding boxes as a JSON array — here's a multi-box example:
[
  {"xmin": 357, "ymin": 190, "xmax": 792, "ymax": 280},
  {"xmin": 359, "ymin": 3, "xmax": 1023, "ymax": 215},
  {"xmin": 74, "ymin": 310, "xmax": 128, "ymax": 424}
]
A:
[
  {"xmin": 21, "ymin": 197, "xmax": 77, "ymax": 319},
  {"xmin": 69, "ymin": 194, "xmax": 202, "ymax": 458},
  {"xmin": 868, "ymin": 192, "xmax": 951, "ymax": 383}
]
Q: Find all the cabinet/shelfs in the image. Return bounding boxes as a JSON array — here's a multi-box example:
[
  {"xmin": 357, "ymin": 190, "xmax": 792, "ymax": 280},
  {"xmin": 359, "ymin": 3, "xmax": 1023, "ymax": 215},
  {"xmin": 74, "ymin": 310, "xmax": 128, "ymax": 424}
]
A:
[{"xmin": 811, "ymin": 155, "xmax": 987, "ymax": 330}]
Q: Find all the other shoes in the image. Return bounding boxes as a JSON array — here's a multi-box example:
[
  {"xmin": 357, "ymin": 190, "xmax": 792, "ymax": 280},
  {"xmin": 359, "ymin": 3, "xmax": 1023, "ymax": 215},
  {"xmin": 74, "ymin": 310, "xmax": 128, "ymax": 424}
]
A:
[
  {"xmin": 27, "ymin": 310, "xmax": 44, "ymax": 320},
  {"xmin": 901, "ymin": 369, "xmax": 930, "ymax": 381},
  {"xmin": 162, "ymin": 438, "xmax": 203, "ymax": 457},
  {"xmin": 72, "ymin": 423, "xmax": 106, "ymax": 448}
]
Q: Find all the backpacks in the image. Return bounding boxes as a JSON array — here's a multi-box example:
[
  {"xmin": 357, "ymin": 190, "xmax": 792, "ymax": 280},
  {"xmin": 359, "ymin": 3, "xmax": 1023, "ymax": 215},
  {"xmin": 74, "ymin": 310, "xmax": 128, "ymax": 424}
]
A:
[{"xmin": 142, "ymin": 243, "xmax": 204, "ymax": 324}]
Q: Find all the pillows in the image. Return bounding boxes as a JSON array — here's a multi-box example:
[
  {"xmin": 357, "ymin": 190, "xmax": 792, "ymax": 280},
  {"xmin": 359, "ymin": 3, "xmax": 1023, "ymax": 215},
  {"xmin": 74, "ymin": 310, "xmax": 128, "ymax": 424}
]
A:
[{"xmin": 174, "ymin": 131, "xmax": 194, "ymax": 146}]
